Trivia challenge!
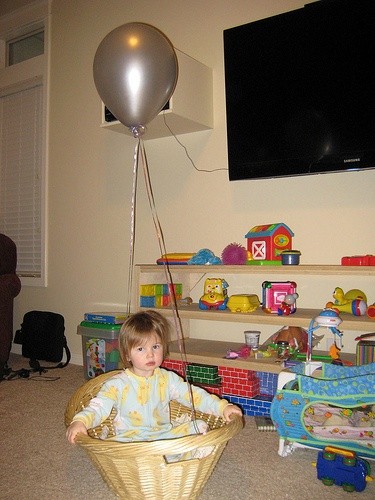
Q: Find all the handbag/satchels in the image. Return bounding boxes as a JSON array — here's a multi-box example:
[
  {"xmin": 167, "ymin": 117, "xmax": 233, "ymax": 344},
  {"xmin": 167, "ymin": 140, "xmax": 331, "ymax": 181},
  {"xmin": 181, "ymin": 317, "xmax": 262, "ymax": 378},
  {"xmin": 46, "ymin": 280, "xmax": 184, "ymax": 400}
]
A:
[{"xmin": 21, "ymin": 310, "xmax": 65, "ymax": 362}]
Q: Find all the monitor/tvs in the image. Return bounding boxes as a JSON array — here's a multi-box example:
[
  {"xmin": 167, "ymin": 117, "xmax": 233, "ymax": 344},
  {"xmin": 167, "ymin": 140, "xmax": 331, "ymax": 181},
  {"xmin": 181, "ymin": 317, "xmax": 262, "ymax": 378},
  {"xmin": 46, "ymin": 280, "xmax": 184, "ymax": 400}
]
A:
[{"xmin": 223, "ymin": 0, "xmax": 375, "ymax": 180}]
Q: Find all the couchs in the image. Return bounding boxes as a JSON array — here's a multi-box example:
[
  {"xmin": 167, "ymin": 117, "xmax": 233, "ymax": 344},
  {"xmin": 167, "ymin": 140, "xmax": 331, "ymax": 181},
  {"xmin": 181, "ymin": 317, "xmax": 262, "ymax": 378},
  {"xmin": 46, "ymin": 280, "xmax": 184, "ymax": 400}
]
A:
[{"xmin": 0, "ymin": 233, "xmax": 22, "ymax": 375}]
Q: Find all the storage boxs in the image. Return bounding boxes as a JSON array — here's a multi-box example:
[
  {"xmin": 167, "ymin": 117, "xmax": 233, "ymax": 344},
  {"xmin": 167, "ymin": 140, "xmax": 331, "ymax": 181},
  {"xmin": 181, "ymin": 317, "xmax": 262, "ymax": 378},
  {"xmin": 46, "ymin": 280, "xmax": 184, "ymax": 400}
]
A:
[
  {"xmin": 84, "ymin": 311, "xmax": 134, "ymax": 326},
  {"xmin": 76, "ymin": 320, "xmax": 127, "ymax": 380}
]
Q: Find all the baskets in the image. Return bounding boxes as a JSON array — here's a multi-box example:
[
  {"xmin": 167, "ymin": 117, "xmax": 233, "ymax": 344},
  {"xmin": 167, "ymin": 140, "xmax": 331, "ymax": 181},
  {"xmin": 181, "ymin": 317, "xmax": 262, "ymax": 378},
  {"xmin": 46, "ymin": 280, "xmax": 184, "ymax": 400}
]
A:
[{"xmin": 64, "ymin": 368, "xmax": 245, "ymax": 500}]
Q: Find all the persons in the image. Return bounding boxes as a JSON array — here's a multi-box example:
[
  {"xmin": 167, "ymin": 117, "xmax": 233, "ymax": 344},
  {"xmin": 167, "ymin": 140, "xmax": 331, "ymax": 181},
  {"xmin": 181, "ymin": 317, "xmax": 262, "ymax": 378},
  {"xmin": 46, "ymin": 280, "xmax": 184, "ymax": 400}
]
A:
[{"xmin": 65, "ymin": 310, "xmax": 243, "ymax": 464}]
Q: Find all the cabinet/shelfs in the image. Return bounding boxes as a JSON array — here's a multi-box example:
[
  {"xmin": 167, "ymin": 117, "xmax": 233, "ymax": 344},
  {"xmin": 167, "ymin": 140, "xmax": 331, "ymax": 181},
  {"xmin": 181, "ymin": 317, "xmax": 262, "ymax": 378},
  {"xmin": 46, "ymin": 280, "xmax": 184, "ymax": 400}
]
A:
[{"xmin": 134, "ymin": 263, "xmax": 375, "ymax": 375}]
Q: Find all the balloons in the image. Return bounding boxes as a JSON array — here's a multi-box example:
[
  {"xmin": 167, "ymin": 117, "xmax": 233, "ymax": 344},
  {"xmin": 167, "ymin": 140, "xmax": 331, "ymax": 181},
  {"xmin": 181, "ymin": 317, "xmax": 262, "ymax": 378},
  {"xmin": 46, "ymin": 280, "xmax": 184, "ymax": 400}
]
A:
[{"xmin": 93, "ymin": 23, "xmax": 177, "ymax": 129}]
